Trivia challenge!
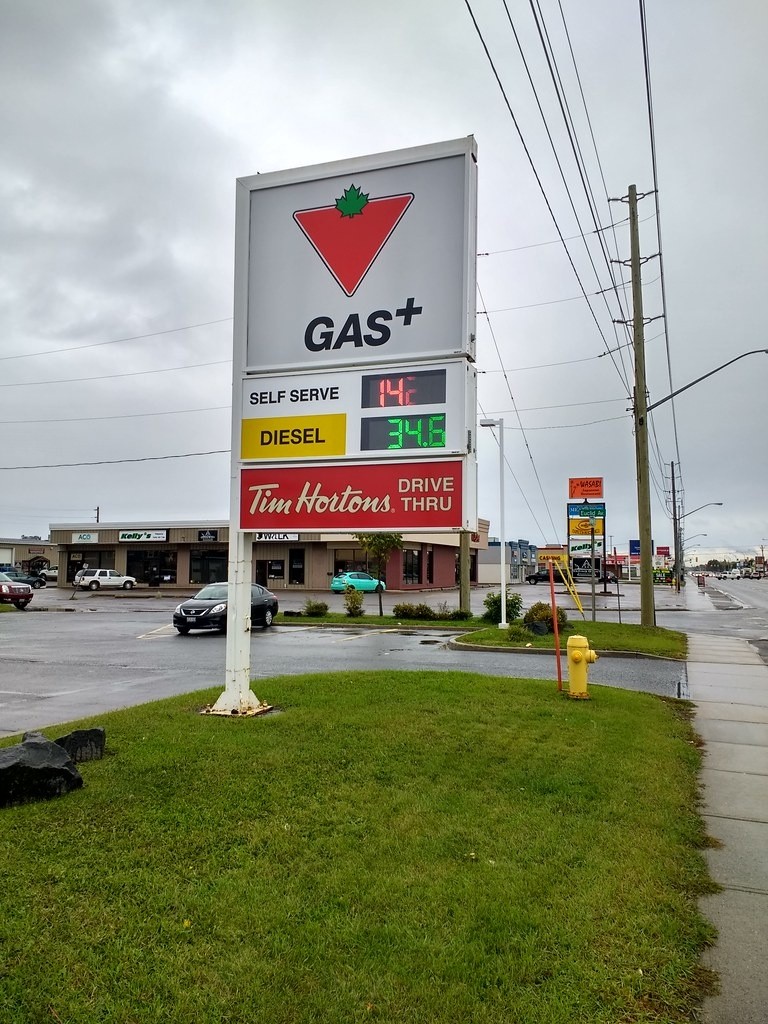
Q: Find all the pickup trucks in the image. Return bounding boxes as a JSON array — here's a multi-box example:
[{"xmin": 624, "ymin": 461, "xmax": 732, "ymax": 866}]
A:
[{"xmin": 526, "ymin": 569, "xmax": 572, "ymax": 585}]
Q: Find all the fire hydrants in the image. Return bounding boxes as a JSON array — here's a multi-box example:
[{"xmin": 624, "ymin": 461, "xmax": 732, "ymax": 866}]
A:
[{"xmin": 566, "ymin": 635, "xmax": 598, "ymax": 701}]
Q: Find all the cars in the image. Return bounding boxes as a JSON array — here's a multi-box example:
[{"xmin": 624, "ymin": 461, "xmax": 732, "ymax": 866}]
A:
[
  {"xmin": 3, "ymin": 571, "xmax": 47, "ymax": 590},
  {"xmin": 689, "ymin": 570, "xmax": 762, "ymax": 581},
  {"xmin": 72, "ymin": 569, "xmax": 137, "ymax": 591},
  {"xmin": 598, "ymin": 571, "xmax": 618, "ymax": 584},
  {"xmin": 37, "ymin": 565, "xmax": 59, "ymax": 582},
  {"xmin": 0, "ymin": 572, "xmax": 34, "ymax": 609},
  {"xmin": 330, "ymin": 571, "xmax": 386, "ymax": 594},
  {"xmin": 154, "ymin": 569, "xmax": 176, "ymax": 583},
  {"xmin": 0, "ymin": 563, "xmax": 17, "ymax": 573},
  {"xmin": 172, "ymin": 582, "xmax": 278, "ymax": 635}
]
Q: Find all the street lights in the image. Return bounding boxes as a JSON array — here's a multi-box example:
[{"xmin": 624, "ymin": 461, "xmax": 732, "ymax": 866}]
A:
[
  {"xmin": 480, "ymin": 417, "xmax": 509, "ymax": 629},
  {"xmin": 673, "ymin": 502, "xmax": 723, "ymax": 579},
  {"xmin": 673, "ymin": 533, "xmax": 707, "ymax": 578}
]
